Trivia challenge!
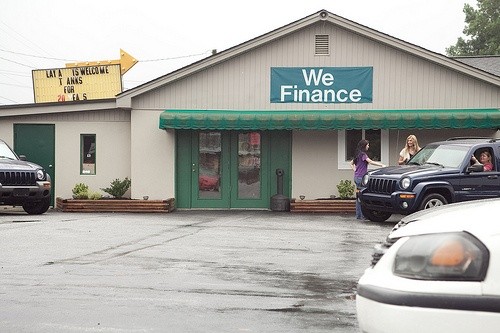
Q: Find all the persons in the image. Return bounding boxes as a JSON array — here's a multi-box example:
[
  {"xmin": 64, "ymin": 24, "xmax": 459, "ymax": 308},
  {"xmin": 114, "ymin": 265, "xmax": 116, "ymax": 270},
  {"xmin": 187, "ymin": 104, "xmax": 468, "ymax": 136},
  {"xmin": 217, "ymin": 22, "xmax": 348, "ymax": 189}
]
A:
[
  {"xmin": 399, "ymin": 135, "xmax": 422, "ymax": 164},
  {"xmin": 472, "ymin": 151, "xmax": 492, "ymax": 171},
  {"xmin": 350, "ymin": 139, "xmax": 386, "ymax": 220}
]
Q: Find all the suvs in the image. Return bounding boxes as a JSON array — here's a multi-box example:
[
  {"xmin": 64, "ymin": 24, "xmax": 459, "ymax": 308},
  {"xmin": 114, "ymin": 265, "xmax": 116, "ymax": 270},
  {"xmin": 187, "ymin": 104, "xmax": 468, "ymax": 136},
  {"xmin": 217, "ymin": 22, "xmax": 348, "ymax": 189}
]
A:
[
  {"xmin": 354, "ymin": 197, "xmax": 500, "ymax": 333},
  {"xmin": 355, "ymin": 135, "xmax": 500, "ymax": 223},
  {"xmin": 0, "ymin": 139, "xmax": 52, "ymax": 215}
]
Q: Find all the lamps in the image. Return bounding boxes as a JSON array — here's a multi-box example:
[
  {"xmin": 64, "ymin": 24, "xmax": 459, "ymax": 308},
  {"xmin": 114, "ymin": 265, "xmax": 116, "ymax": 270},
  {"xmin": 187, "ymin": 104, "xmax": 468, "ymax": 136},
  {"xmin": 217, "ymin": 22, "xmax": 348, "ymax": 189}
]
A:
[
  {"xmin": 143, "ymin": 196, "xmax": 149, "ymax": 200},
  {"xmin": 299, "ymin": 196, "xmax": 305, "ymax": 200},
  {"xmin": 330, "ymin": 195, "xmax": 335, "ymax": 199}
]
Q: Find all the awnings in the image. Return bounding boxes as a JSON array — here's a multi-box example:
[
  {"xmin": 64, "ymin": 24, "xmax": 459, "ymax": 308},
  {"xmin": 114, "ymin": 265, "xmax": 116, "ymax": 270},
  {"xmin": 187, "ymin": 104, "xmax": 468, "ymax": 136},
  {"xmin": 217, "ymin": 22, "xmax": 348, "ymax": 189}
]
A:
[{"xmin": 159, "ymin": 109, "xmax": 500, "ymax": 129}]
